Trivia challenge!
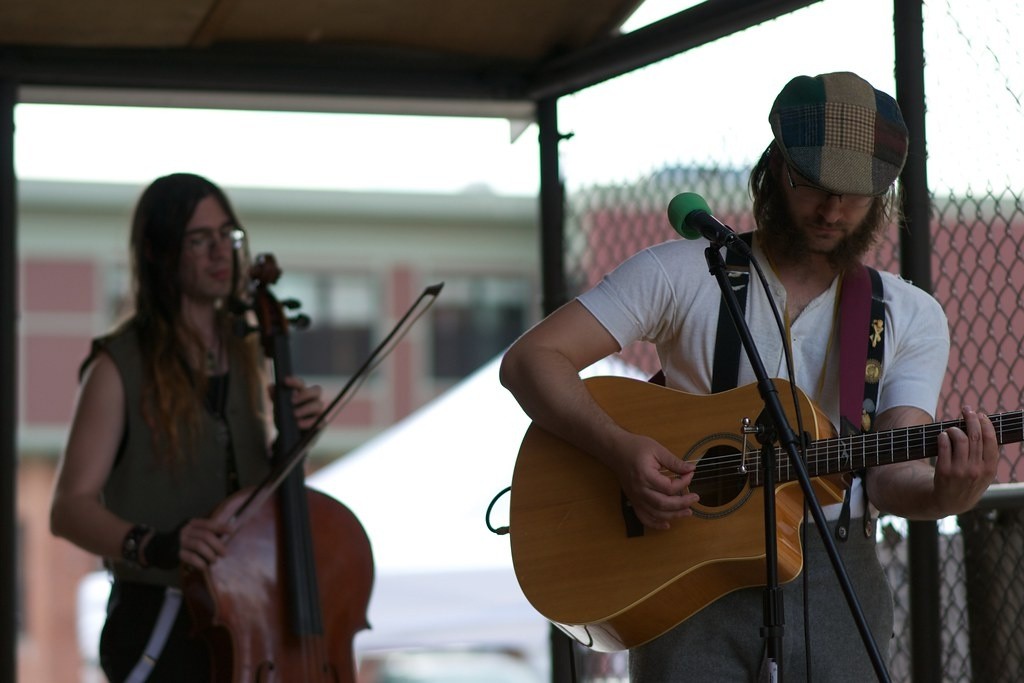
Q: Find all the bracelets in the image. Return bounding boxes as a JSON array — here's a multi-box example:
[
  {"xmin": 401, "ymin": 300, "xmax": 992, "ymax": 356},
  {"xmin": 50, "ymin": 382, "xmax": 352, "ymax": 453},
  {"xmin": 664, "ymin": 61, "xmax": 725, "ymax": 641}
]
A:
[{"xmin": 121, "ymin": 526, "xmax": 148, "ymax": 564}]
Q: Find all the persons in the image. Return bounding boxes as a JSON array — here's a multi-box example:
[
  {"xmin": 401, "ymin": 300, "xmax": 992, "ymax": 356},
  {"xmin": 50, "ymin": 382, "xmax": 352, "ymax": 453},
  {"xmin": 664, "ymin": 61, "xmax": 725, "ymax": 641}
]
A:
[
  {"xmin": 499, "ymin": 73, "xmax": 999, "ymax": 683},
  {"xmin": 50, "ymin": 172, "xmax": 325, "ymax": 683}
]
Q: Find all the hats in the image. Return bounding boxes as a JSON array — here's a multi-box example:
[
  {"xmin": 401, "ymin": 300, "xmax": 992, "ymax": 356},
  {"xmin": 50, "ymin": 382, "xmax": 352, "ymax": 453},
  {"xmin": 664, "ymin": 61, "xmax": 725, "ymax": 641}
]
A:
[{"xmin": 769, "ymin": 72, "xmax": 909, "ymax": 195}]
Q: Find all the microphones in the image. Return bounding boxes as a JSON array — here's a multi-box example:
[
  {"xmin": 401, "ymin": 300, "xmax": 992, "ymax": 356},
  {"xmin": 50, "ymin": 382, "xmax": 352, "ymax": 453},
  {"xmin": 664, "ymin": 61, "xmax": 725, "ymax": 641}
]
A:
[{"xmin": 667, "ymin": 192, "xmax": 754, "ymax": 262}]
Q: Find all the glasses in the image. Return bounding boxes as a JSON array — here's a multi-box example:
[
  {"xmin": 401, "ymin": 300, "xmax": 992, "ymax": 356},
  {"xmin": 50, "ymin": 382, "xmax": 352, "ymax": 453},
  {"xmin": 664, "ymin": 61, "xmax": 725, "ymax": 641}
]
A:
[
  {"xmin": 786, "ymin": 164, "xmax": 874, "ymax": 208},
  {"xmin": 182, "ymin": 227, "xmax": 244, "ymax": 254}
]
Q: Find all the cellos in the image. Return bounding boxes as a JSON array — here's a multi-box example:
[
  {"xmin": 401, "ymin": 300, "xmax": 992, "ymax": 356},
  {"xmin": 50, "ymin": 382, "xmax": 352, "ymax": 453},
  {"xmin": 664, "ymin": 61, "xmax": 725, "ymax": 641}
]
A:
[{"xmin": 193, "ymin": 250, "xmax": 376, "ymax": 683}]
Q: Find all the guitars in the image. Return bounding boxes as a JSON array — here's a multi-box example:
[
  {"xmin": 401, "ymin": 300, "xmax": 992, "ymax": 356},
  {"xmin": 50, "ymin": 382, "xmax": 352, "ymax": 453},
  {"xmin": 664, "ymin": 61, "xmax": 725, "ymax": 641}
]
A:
[{"xmin": 506, "ymin": 369, "xmax": 1024, "ymax": 651}]
